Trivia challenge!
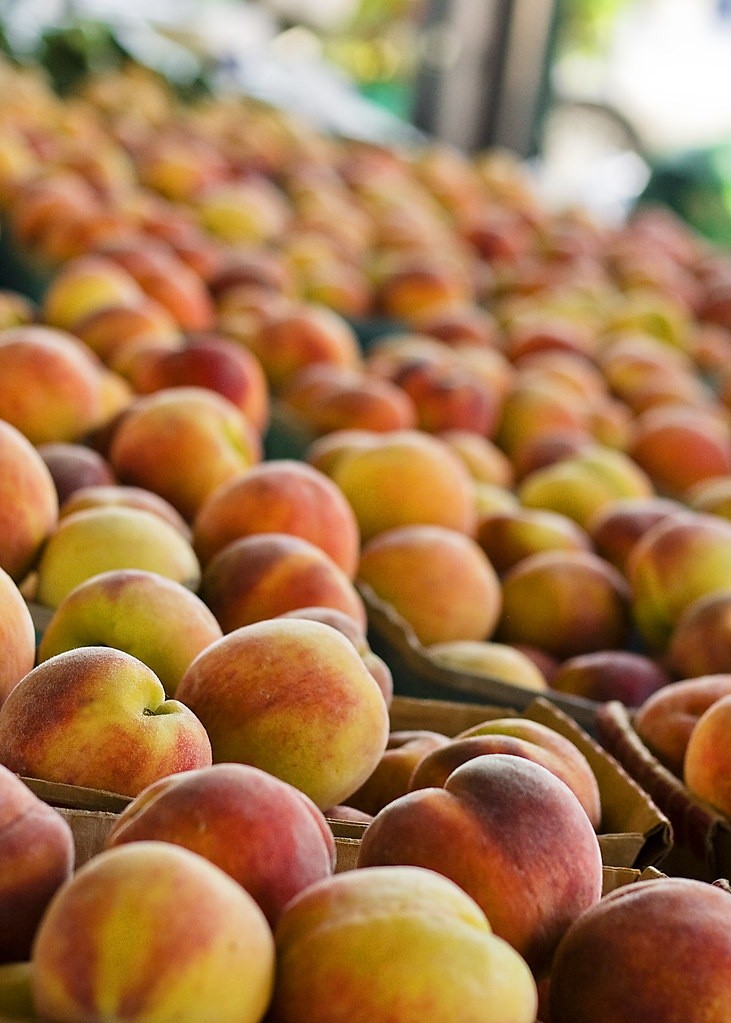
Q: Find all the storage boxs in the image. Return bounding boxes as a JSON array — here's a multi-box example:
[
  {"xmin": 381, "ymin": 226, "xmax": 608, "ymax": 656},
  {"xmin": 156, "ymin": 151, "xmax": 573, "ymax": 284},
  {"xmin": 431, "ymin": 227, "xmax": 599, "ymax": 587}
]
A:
[{"xmin": 19, "ymin": 577, "xmax": 731, "ymax": 898}]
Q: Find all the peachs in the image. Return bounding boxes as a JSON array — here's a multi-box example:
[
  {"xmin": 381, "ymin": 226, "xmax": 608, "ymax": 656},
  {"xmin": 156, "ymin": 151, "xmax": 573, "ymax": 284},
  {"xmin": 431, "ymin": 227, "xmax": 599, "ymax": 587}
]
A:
[{"xmin": 0, "ymin": 54, "xmax": 731, "ymax": 1023}]
